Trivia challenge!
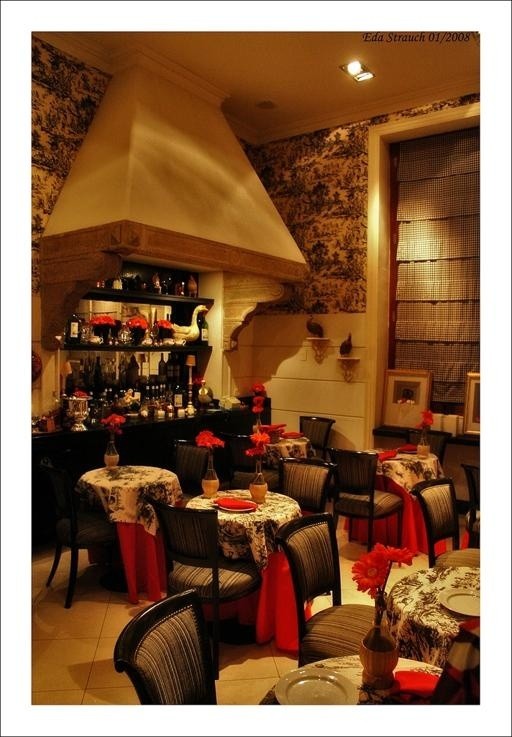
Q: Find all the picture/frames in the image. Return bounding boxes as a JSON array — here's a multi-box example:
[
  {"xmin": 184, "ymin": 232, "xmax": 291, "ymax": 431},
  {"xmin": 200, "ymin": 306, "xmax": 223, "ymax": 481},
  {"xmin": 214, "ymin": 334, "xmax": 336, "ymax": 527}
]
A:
[
  {"xmin": 381, "ymin": 368, "xmax": 431, "ymax": 429},
  {"xmin": 462, "ymin": 373, "xmax": 480, "ymax": 435}
]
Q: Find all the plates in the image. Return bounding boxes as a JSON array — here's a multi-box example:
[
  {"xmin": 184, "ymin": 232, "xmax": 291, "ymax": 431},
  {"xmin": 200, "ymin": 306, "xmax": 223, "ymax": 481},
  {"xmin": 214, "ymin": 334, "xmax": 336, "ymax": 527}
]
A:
[
  {"xmin": 216, "ymin": 499, "xmax": 256, "ymax": 512},
  {"xmin": 404, "ymin": 450, "xmax": 418, "ymax": 453},
  {"xmin": 276, "ymin": 668, "xmax": 360, "ymax": 704},
  {"xmin": 389, "ymin": 455, "xmax": 402, "ymax": 459},
  {"xmin": 438, "ymin": 588, "xmax": 480, "ymax": 617}
]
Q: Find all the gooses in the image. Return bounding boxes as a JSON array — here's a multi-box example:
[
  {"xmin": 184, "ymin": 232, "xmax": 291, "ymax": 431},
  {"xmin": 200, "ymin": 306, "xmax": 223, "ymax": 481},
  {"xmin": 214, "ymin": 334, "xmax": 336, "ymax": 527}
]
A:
[{"xmin": 171, "ymin": 304, "xmax": 208, "ymax": 341}]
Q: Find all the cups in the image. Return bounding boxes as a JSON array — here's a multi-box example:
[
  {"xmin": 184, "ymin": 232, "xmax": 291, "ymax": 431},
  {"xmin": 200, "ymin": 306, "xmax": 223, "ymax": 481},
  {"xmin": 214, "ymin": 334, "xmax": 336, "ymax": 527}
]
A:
[{"xmin": 430, "ymin": 414, "xmax": 466, "ymax": 437}]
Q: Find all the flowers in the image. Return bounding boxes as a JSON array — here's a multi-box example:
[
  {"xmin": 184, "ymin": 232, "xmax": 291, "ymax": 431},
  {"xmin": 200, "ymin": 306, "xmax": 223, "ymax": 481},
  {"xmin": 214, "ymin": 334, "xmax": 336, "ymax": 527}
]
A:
[
  {"xmin": 101, "ymin": 410, "xmax": 127, "ymax": 435},
  {"xmin": 415, "ymin": 407, "xmax": 432, "ymax": 434},
  {"xmin": 89, "ymin": 310, "xmax": 115, "ymax": 329}
]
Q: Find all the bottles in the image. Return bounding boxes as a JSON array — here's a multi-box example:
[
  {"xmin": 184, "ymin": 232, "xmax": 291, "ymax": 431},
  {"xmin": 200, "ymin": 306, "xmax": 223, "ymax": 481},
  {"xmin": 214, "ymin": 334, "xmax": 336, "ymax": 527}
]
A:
[{"xmin": 63, "ymin": 270, "xmax": 214, "ymax": 432}]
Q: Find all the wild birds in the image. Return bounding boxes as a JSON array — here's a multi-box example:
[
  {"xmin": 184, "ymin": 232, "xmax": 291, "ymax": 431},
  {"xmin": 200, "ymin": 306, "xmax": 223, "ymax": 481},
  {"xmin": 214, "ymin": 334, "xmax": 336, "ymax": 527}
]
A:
[
  {"xmin": 307, "ymin": 315, "xmax": 325, "ymax": 338},
  {"xmin": 339, "ymin": 331, "xmax": 353, "ymax": 357}
]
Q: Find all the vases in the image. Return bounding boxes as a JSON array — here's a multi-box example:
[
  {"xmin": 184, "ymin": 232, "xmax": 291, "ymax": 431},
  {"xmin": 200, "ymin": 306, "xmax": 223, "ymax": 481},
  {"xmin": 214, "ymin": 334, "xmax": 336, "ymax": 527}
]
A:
[{"xmin": 105, "ymin": 438, "xmax": 120, "ymax": 471}]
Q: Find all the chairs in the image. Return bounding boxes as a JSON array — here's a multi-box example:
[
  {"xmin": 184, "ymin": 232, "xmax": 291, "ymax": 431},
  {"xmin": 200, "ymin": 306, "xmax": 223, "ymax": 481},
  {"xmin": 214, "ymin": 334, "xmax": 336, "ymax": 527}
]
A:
[
  {"xmin": 41, "ymin": 456, "xmax": 118, "ymax": 609},
  {"xmin": 137, "ymin": 494, "xmax": 262, "ymax": 680},
  {"xmin": 114, "ymin": 589, "xmax": 218, "ymax": 705}
]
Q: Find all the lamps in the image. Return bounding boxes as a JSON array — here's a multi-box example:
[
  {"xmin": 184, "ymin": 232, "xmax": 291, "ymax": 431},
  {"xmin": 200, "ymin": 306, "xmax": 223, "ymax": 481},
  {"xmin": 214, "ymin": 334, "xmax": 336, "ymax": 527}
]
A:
[
  {"xmin": 185, "ymin": 354, "xmax": 197, "ymax": 406},
  {"xmin": 157, "ymin": 317, "xmax": 172, "ymax": 332},
  {"xmin": 125, "ymin": 311, "xmax": 152, "ymax": 333}
]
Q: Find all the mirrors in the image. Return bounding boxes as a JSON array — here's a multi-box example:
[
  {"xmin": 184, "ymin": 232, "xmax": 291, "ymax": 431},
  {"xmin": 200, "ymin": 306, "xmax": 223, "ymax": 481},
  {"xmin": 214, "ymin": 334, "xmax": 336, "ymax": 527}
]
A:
[{"xmin": 64, "ymin": 300, "xmax": 172, "ymax": 383}]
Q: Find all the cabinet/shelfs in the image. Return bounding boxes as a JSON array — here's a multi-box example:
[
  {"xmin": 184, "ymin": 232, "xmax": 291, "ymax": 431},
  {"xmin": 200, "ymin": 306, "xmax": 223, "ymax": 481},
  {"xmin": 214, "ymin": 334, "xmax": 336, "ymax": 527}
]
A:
[{"xmin": 63, "ymin": 286, "xmax": 214, "ymax": 355}]
